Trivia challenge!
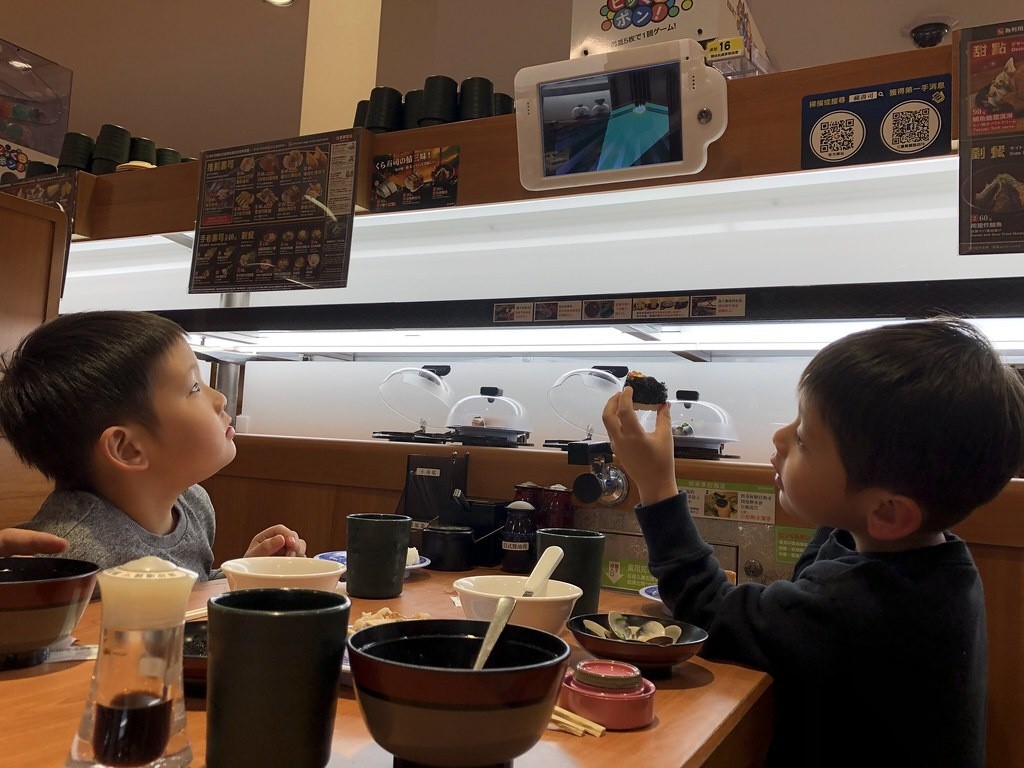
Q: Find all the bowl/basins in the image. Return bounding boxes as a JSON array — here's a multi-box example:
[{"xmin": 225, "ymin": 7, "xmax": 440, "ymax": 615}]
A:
[
  {"xmin": 451, "ymin": 576, "xmax": 582, "ymax": 637},
  {"xmin": 566, "ymin": 613, "xmax": 709, "ymax": 681},
  {"xmin": 219, "ymin": 556, "xmax": 347, "ymax": 592},
  {"xmin": 344, "ymin": 618, "xmax": 572, "ymax": 767},
  {"xmin": 183, "ymin": 619, "xmax": 207, "ymax": 677},
  {"xmin": 0, "ymin": 557, "xmax": 101, "ymax": 671}
]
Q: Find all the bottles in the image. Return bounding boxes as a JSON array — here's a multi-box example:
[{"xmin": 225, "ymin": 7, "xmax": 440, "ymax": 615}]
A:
[
  {"xmin": 65, "ymin": 554, "xmax": 199, "ymax": 768},
  {"xmin": 502, "ymin": 501, "xmax": 537, "ymax": 574}
]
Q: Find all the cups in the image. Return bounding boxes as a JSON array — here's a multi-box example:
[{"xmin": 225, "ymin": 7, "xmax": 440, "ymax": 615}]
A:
[
  {"xmin": 204, "ymin": 586, "xmax": 352, "ymax": 768},
  {"xmin": 536, "ymin": 528, "xmax": 607, "ymax": 616},
  {"xmin": 346, "ymin": 512, "xmax": 412, "ymax": 601}
]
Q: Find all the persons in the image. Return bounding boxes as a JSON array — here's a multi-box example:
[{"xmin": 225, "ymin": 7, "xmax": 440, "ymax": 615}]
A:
[
  {"xmin": 603, "ymin": 320, "xmax": 1022, "ymax": 768},
  {"xmin": 0, "ymin": 311, "xmax": 306, "ymax": 589}
]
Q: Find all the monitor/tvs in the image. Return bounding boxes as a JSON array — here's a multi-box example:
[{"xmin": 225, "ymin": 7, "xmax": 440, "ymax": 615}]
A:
[{"xmin": 515, "ymin": 38, "xmax": 727, "ymax": 193}]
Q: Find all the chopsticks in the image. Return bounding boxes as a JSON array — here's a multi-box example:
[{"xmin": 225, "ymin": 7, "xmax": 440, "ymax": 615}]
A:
[
  {"xmin": 185, "ymin": 607, "xmax": 207, "ymax": 620},
  {"xmin": 552, "ymin": 706, "xmax": 606, "ymax": 738}
]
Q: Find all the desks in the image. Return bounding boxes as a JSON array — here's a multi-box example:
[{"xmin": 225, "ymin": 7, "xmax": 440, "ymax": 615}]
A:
[{"xmin": 0, "ymin": 571, "xmax": 769, "ymax": 768}]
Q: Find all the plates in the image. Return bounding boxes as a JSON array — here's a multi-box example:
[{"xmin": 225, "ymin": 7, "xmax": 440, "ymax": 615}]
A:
[
  {"xmin": 313, "ymin": 551, "xmax": 432, "ymax": 580},
  {"xmin": 638, "ymin": 585, "xmax": 673, "ymax": 617}
]
